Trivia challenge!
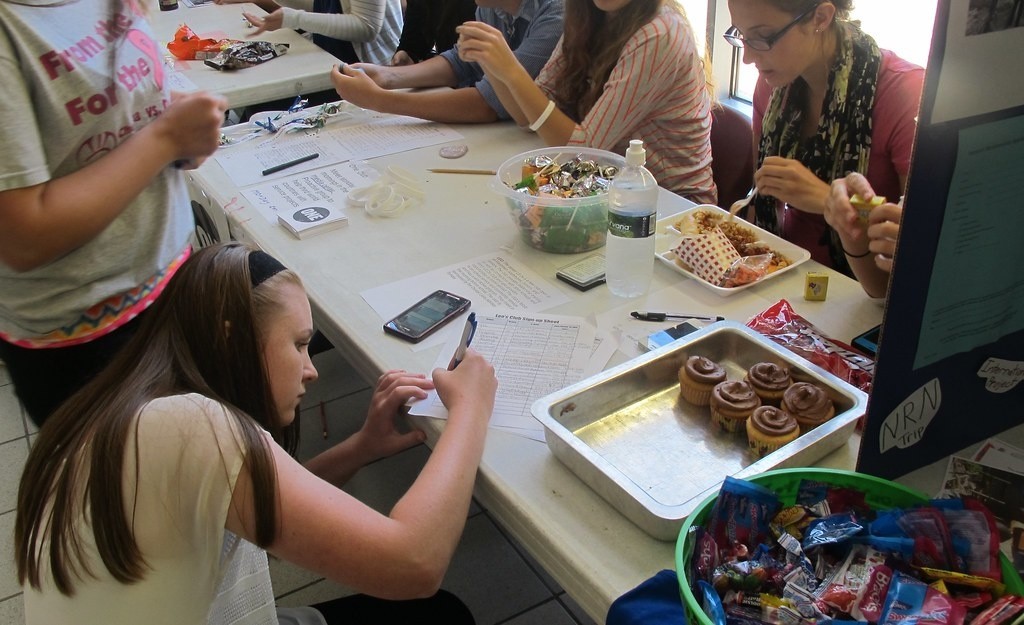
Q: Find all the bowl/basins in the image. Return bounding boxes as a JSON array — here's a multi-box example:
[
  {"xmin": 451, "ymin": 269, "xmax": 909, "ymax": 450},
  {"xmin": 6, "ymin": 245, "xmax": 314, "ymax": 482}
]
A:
[
  {"xmin": 673, "ymin": 468, "xmax": 1024, "ymax": 625},
  {"xmin": 488, "ymin": 146, "xmax": 625, "ymax": 254}
]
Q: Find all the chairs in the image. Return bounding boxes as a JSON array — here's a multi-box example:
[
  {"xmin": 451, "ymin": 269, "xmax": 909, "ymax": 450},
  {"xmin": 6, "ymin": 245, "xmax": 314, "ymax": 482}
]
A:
[{"xmin": 709, "ymin": 100, "xmax": 758, "ymax": 224}]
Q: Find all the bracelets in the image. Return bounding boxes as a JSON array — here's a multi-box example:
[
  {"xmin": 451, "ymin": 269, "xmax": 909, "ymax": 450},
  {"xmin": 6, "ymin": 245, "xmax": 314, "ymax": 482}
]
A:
[
  {"xmin": 528, "ymin": 100, "xmax": 555, "ymax": 131},
  {"xmin": 842, "ymin": 248, "xmax": 871, "ymax": 258}
]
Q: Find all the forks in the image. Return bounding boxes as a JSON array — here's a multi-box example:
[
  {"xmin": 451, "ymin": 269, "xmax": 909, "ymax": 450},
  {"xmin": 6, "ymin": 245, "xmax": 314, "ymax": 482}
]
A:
[{"xmin": 730, "ymin": 187, "xmax": 758, "ymax": 219}]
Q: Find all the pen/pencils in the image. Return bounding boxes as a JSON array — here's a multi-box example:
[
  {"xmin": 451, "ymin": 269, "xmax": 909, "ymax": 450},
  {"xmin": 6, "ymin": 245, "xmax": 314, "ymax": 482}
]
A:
[
  {"xmin": 320, "ymin": 401, "xmax": 328, "ymax": 438},
  {"xmin": 453, "ymin": 312, "xmax": 477, "ymax": 368},
  {"xmin": 262, "ymin": 153, "xmax": 319, "ymax": 175},
  {"xmin": 631, "ymin": 311, "xmax": 726, "ymax": 322},
  {"xmin": 425, "ymin": 168, "xmax": 496, "ymax": 174}
]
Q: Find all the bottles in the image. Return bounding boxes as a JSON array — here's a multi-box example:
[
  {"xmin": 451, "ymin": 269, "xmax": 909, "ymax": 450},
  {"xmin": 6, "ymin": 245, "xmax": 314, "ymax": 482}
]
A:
[
  {"xmin": 604, "ymin": 139, "xmax": 660, "ymax": 298},
  {"xmin": 158, "ymin": 0, "xmax": 178, "ymax": 11}
]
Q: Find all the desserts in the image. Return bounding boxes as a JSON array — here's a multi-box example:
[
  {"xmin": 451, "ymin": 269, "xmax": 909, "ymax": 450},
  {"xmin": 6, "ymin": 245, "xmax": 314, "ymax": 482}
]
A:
[{"xmin": 678, "ymin": 355, "xmax": 834, "ymax": 459}]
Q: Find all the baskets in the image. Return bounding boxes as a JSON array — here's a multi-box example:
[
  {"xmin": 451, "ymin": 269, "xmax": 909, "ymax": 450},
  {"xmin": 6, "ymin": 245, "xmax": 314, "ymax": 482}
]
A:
[{"xmin": 676, "ymin": 467, "xmax": 1023, "ymax": 625}]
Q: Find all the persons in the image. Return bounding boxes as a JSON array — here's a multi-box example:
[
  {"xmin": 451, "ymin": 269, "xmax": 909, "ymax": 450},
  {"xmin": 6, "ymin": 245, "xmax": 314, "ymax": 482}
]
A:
[
  {"xmin": 330, "ymin": 0, "xmax": 565, "ymax": 124},
  {"xmin": 723, "ymin": 0, "xmax": 925, "ymax": 282},
  {"xmin": 824, "ymin": 172, "xmax": 902, "ymax": 299},
  {"xmin": 455, "ymin": 0, "xmax": 718, "ymax": 207},
  {"xmin": 238, "ymin": 0, "xmax": 404, "ymax": 124},
  {"xmin": 14, "ymin": 241, "xmax": 498, "ymax": 625},
  {"xmin": 391, "ymin": 0, "xmax": 478, "ymax": 66},
  {"xmin": 0, "ymin": 0, "xmax": 229, "ymax": 432}
]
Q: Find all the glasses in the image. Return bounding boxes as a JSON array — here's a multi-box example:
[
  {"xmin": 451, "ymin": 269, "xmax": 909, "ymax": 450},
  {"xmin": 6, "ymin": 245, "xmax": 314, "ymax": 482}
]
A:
[{"xmin": 724, "ymin": 0, "xmax": 824, "ymax": 51}]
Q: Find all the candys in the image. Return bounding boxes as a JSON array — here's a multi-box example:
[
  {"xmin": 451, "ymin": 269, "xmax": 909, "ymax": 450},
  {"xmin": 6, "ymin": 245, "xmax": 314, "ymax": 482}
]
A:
[
  {"xmin": 686, "ymin": 477, "xmax": 1024, "ymax": 625},
  {"xmin": 506, "ymin": 152, "xmax": 622, "ymax": 253}
]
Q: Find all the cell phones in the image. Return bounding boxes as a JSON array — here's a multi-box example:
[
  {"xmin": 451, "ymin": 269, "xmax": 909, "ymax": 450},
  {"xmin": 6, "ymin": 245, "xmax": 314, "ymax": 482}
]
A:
[
  {"xmin": 852, "ymin": 324, "xmax": 881, "ymax": 357},
  {"xmin": 383, "ymin": 289, "xmax": 471, "ymax": 343}
]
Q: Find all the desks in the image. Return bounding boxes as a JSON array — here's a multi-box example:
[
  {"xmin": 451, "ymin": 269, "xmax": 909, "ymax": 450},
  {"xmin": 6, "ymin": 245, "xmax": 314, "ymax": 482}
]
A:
[
  {"xmin": 181, "ymin": 88, "xmax": 884, "ymax": 624},
  {"xmin": 147, "ymin": 0, "xmax": 346, "ymax": 109}
]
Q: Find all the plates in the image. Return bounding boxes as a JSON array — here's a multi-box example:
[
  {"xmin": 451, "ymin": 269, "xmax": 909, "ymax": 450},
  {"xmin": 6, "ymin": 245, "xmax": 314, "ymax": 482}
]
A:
[{"xmin": 647, "ymin": 203, "xmax": 811, "ymax": 298}]
